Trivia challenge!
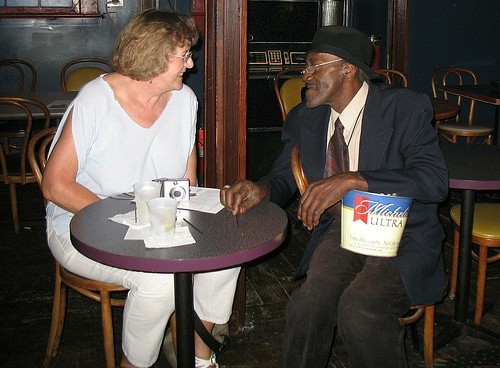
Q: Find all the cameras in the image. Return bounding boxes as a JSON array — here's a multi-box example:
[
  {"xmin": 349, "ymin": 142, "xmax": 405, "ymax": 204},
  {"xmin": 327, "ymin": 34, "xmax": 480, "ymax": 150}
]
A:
[{"xmin": 152, "ymin": 179, "xmax": 190, "ymax": 201}]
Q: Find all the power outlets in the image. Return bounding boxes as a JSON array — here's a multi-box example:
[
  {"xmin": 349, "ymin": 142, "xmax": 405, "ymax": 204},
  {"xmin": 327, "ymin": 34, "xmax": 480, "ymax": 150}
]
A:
[{"xmin": 107, "ymin": 0, "xmax": 123, "ymax": 7}]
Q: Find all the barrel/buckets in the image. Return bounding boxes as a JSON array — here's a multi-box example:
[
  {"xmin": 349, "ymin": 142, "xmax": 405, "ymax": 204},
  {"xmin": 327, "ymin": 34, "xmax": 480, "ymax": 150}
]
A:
[{"xmin": 339, "ymin": 189, "xmax": 413, "ymax": 258}]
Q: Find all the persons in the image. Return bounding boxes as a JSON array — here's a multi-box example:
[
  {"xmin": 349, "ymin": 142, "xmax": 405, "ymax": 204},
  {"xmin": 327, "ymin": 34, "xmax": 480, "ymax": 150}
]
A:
[
  {"xmin": 220, "ymin": 26, "xmax": 449, "ymax": 368},
  {"xmin": 42, "ymin": 7, "xmax": 241, "ymax": 368}
]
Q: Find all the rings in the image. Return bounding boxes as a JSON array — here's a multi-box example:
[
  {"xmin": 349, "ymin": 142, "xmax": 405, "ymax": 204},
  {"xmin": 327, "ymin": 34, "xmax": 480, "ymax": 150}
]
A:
[{"xmin": 223, "ymin": 185, "xmax": 231, "ymax": 189}]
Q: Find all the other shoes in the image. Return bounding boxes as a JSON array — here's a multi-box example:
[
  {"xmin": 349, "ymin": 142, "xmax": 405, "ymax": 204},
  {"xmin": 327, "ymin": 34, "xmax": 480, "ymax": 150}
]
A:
[{"xmin": 196, "ymin": 354, "xmax": 219, "ymax": 368}]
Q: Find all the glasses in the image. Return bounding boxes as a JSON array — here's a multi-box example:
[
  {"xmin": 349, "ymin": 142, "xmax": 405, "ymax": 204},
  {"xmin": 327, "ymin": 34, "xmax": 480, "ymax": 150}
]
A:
[
  {"xmin": 300, "ymin": 58, "xmax": 345, "ymax": 76},
  {"xmin": 173, "ymin": 51, "xmax": 194, "ymax": 60}
]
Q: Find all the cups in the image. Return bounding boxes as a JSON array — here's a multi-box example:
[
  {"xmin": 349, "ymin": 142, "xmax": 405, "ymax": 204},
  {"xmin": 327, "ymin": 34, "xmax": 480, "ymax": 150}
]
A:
[
  {"xmin": 146, "ymin": 197, "xmax": 178, "ymax": 242},
  {"xmin": 132, "ymin": 180, "xmax": 162, "ymax": 225}
]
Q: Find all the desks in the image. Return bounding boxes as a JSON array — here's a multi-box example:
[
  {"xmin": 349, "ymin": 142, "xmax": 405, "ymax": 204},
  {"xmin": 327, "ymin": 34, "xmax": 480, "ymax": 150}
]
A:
[
  {"xmin": 420, "ymin": 143, "xmax": 500, "ymax": 360},
  {"xmin": 70, "ymin": 187, "xmax": 288, "ymax": 368},
  {"xmin": 438, "ymin": 85, "xmax": 500, "ymax": 144},
  {"xmin": 0, "ymin": 90, "xmax": 79, "ymax": 120},
  {"xmin": 433, "ymin": 99, "xmax": 458, "ymax": 120}
]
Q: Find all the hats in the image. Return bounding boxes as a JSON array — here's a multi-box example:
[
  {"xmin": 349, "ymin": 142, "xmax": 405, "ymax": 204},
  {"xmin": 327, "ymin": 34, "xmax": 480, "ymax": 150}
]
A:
[{"xmin": 303, "ymin": 25, "xmax": 382, "ymax": 80}]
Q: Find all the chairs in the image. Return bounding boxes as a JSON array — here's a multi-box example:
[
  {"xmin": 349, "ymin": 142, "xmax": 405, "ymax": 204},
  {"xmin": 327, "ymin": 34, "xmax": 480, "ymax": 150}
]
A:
[
  {"xmin": 430, "ymin": 67, "xmax": 494, "ymax": 145},
  {"xmin": 292, "ymin": 146, "xmax": 448, "ymax": 368},
  {"xmin": 0, "ymin": 59, "xmax": 50, "ymax": 234},
  {"xmin": 273, "ymin": 67, "xmax": 306, "ymax": 121},
  {"xmin": 61, "ymin": 58, "xmax": 113, "ymax": 91},
  {"xmin": 448, "ymin": 203, "xmax": 500, "ymax": 325},
  {"xmin": 28, "ymin": 126, "xmax": 177, "ymax": 368}
]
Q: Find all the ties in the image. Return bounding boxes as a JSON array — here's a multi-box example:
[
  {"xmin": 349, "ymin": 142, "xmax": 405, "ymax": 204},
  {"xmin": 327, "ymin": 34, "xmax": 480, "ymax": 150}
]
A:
[{"xmin": 325, "ymin": 118, "xmax": 350, "ymax": 225}]
